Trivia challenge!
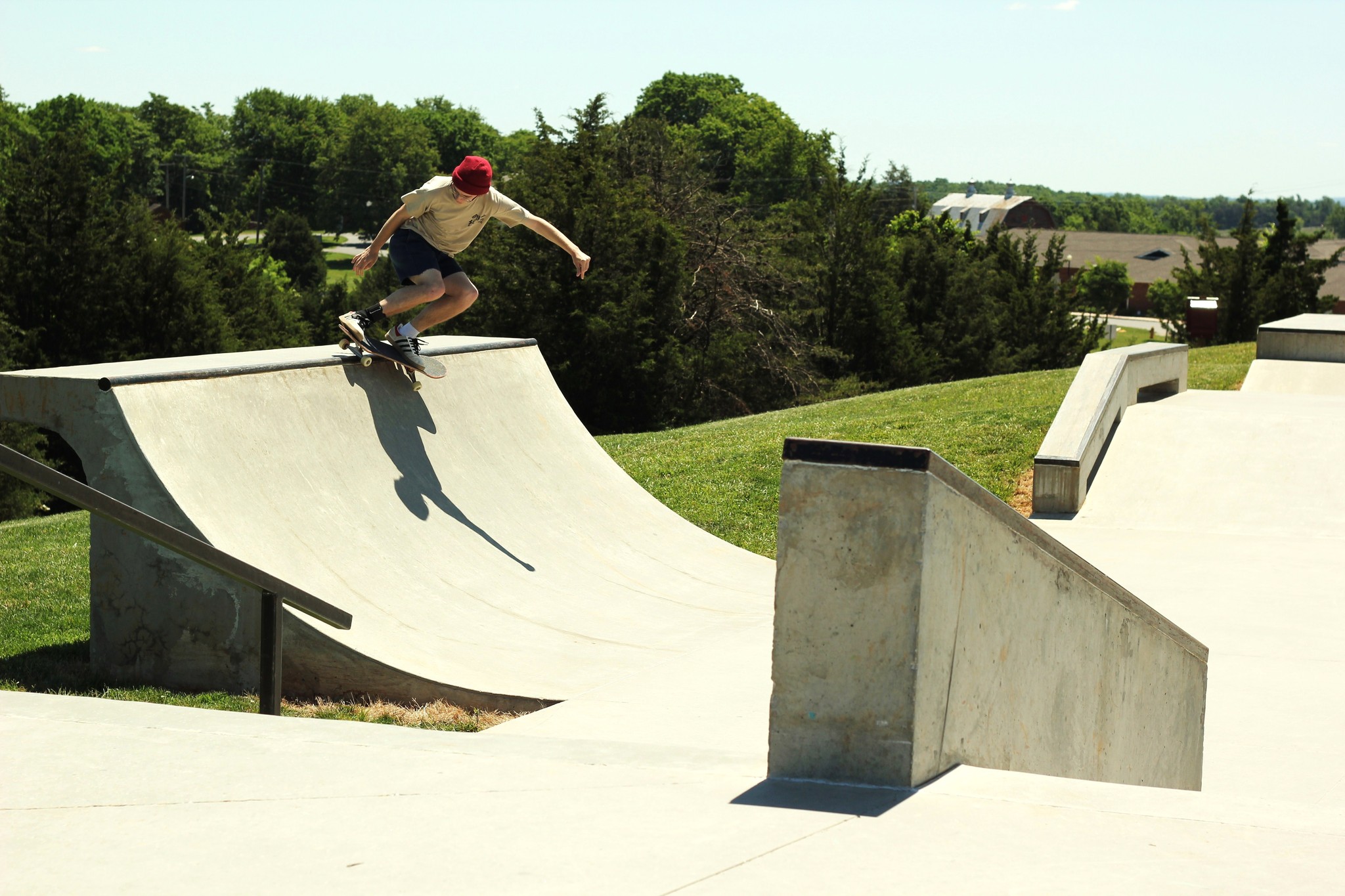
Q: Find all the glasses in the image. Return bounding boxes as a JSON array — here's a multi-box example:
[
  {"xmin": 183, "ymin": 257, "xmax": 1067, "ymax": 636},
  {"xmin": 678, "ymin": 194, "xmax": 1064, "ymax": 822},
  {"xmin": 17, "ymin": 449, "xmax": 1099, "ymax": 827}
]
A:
[{"xmin": 449, "ymin": 181, "xmax": 478, "ymax": 203}]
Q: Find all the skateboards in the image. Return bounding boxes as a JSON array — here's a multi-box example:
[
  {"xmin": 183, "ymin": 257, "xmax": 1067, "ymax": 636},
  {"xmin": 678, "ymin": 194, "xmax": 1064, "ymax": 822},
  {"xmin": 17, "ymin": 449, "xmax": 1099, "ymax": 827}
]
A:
[{"xmin": 339, "ymin": 324, "xmax": 447, "ymax": 391}]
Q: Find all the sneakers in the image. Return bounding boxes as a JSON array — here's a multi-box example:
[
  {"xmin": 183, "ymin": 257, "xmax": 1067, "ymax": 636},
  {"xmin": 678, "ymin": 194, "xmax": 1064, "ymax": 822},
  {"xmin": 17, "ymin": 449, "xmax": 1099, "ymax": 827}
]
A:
[
  {"xmin": 385, "ymin": 323, "xmax": 426, "ymax": 371},
  {"xmin": 339, "ymin": 311, "xmax": 377, "ymax": 341}
]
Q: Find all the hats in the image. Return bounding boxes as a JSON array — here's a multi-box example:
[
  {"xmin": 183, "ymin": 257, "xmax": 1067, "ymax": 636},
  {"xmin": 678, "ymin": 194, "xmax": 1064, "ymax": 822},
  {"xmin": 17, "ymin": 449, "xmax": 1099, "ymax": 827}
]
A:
[{"xmin": 452, "ymin": 156, "xmax": 493, "ymax": 195}]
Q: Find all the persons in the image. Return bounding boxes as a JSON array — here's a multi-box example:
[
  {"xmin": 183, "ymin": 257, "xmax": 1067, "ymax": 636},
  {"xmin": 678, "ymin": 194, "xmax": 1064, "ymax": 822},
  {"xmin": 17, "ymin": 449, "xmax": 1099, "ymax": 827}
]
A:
[{"xmin": 338, "ymin": 156, "xmax": 590, "ymax": 372}]
